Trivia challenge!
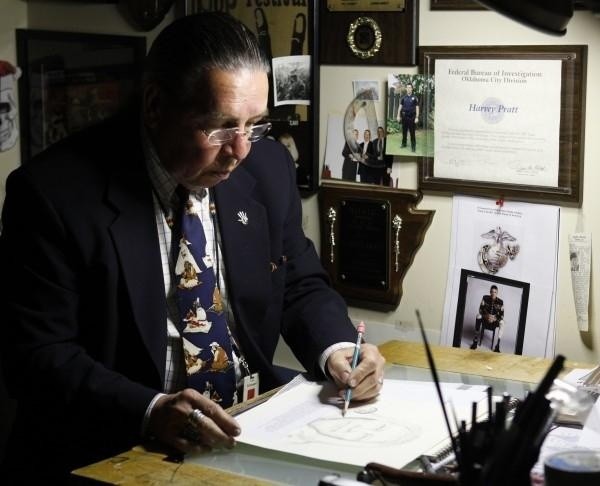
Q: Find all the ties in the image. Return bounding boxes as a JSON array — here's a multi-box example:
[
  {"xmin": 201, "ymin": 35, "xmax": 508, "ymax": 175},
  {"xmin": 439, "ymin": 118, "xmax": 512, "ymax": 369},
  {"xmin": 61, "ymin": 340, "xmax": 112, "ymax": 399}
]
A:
[{"xmin": 173, "ymin": 183, "xmax": 236, "ymax": 410}]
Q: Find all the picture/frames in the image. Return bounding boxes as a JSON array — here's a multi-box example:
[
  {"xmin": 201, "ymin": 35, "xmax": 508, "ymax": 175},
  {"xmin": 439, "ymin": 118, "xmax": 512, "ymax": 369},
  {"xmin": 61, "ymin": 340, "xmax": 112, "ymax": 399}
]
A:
[
  {"xmin": 312, "ymin": 59, "xmax": 427, "ymax": 199},
  {"xmin": 415, "ymin": 44, "xmax": 587, "ymax": 211},
  {"xmin": 173, "ymin": 0, "xmax": 319, "ymax": 199},
  {"xmin": 314, "ymin": 182, "xmax": 436, "ymax": 317},
  {"xmin": 15, "ymin": 27, "xmax": 148, "ymax": 165}
]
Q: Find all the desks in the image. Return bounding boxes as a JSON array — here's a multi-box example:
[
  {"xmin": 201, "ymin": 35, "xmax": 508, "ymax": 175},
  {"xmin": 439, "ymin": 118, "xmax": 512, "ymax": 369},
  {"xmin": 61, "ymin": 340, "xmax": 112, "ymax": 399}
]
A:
[{"xmin": 70, "ymin": 340, "xmax": 599, "ymax": 486}]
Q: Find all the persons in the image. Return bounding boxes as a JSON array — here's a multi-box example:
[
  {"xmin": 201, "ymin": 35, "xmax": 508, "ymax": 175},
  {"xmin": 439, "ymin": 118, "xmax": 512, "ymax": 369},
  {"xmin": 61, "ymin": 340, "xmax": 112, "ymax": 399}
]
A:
[
  {"xmin": 570, "ymin": 253, "xmax": 579, "ymax": 271},
  {"xmin": 469, "ymin": 286, "xmax": 505, "ymax": 353},
  {"xmin": 395, "ymin": 82, "xmax": 420, "ymax": 154},
  {"xmin": 284, "ymin": 69, "xmax": 305, "ymax": 101},
  {"xmin": 343, "ymin": 126, "xmax": 394, "ymax": 187},
  {"xmin": 0, "ymin": 10, "xmax": 387, "ymax": 486}
]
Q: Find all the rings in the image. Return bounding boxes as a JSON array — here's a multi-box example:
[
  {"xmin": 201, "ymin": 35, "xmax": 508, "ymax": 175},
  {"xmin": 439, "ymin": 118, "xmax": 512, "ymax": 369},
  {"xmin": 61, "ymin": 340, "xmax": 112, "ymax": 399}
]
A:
[{"xmin": 185, "ymin": 408, "xmax": 205, "ymax": 429}]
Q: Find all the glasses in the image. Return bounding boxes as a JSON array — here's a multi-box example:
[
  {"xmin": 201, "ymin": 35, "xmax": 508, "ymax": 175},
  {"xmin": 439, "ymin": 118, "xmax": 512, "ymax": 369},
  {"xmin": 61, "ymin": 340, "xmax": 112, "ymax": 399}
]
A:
[{"xmin": 196, "ymin": 118, "xmax": 273, "ymax": 146}]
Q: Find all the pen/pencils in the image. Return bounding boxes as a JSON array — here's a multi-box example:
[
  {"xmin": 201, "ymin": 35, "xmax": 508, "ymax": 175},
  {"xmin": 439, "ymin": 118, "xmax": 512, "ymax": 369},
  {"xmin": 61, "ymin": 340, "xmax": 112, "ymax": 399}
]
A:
[{"xmin": 342, "ymin": 321, "xmax": 366, "ymax": 417}]
